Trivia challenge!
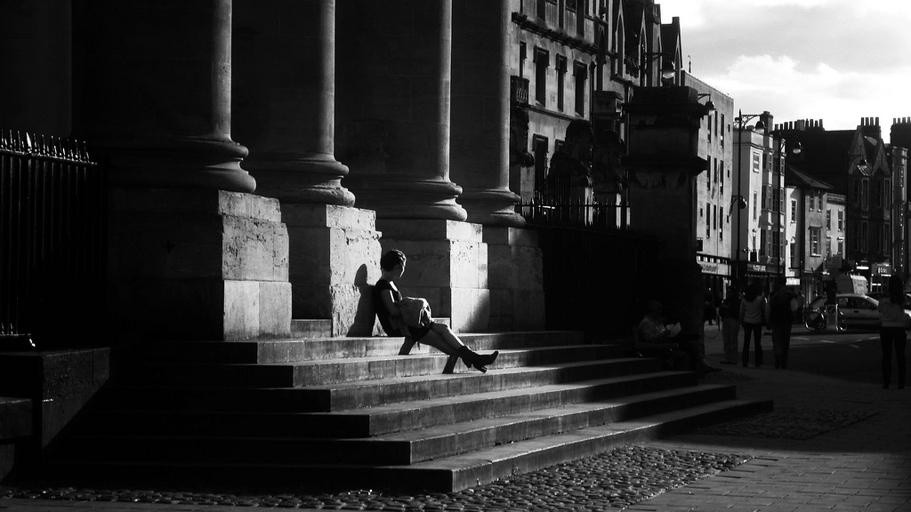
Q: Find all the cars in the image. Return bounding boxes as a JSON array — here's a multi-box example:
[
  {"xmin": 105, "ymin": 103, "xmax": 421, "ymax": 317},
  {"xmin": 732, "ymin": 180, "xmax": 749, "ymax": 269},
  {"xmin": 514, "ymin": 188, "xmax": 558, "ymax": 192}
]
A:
[
  {"xmin": 867, "ymin": 292, "xmax": 911, "ymax": 308},
  {"xmin": 809, "ymin": 293, "xmax": 910, "ymax": 331}
]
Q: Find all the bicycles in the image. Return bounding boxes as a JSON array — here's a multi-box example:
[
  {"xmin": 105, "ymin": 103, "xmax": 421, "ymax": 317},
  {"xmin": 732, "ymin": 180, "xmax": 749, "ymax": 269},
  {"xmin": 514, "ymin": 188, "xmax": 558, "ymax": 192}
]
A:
[{"xmin": 803, "ymin": 304, "xmax": 850, "ymax": 334}]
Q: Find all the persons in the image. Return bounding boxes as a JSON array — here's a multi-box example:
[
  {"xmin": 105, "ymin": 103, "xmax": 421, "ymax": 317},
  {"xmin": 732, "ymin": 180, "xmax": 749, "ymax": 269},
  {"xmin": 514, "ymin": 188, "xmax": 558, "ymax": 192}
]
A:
[
  {"xmin": 373, "ymin": 249, "xmax": 498, "ymax": 375},
  {"xmin": 632, "ymin": 274, "xmax": 837, "ymax": 376},
  {"xmin": 876, "ymin": 274, "xmax": 910, "ymax": 390}
]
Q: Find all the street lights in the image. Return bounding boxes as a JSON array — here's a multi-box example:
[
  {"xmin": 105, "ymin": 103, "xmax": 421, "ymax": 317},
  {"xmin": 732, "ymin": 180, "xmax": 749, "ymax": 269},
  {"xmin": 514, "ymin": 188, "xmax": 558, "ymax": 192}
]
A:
[
  {"xmin": 621, "ymin": 47, "xmax": 679, "ymax": 230},
  {"xmin": 737, "ymin": 106, "xmax": 779, "ymax": 277},
  {"xmin": 778, "ymin": 143, "xmax": 812, "ymax": 279}
]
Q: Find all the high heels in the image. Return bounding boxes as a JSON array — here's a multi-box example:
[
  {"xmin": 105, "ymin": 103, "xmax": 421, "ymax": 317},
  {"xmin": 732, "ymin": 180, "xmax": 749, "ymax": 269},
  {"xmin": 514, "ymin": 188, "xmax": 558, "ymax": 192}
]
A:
[{"xmin": 456, "ymin": 346, "xmax": 498, "ymax": 373}]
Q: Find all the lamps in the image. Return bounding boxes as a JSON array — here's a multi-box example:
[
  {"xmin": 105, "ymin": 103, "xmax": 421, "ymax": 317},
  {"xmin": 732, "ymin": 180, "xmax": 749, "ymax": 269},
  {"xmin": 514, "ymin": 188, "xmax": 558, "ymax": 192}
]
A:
[
  {"xmin": 725, "ymin": 192, "xmax": 747, "ymax": 225},
  {"xmin": 643, "ymin": 50, "xmax": 677, "ymax": 80}
]
